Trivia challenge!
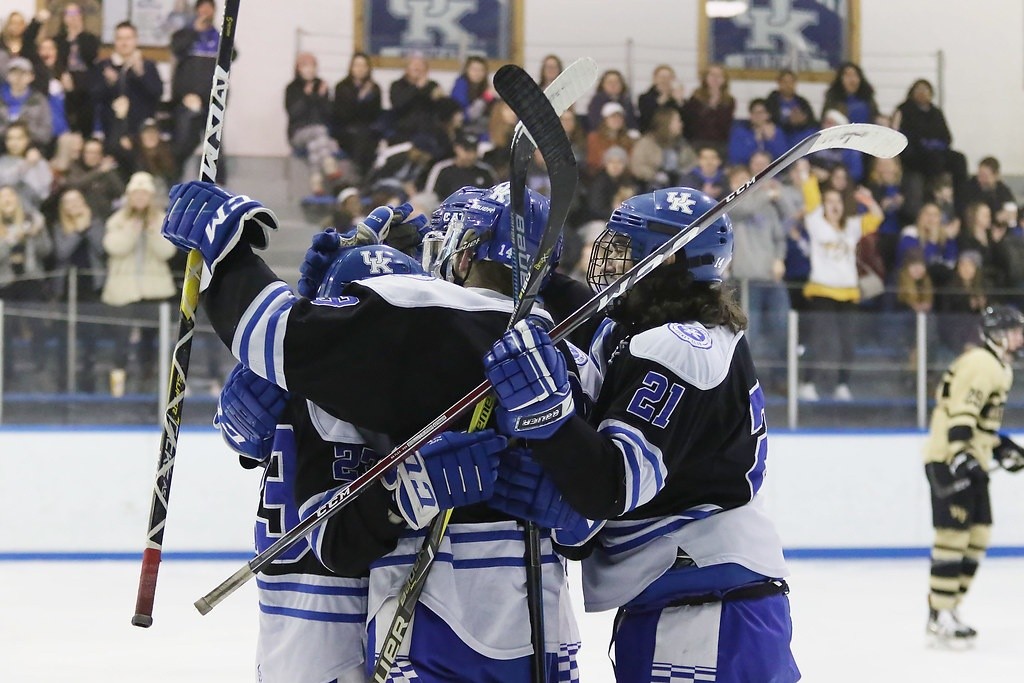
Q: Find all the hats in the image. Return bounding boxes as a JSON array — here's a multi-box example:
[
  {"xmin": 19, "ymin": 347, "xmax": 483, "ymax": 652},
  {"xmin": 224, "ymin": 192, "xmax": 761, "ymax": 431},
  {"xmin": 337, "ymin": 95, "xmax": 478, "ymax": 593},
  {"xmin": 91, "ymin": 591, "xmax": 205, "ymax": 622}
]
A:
[
  {"xmin": 601, "ymin": 102, "xmax": 624, "ymax": 117},
  {"xmin": 8, "ymin": 58, "xmax": 32, "ymax": 72},
  {"xmin": 456, "ymin": 134, "xmax": 480, "ymax": 150}
]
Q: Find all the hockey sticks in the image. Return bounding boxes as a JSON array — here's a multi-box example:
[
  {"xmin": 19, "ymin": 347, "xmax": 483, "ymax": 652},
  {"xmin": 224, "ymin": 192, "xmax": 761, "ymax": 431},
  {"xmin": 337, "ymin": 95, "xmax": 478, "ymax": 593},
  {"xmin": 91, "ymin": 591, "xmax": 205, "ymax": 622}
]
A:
[
  {"xmin": 370, "ymin": 63, "xmax": 579, "ymax": 683},
  {"xmin": 508, "ymin": 54, "xmax": 603, "ymax": 683},
  {"xmin": 925, "ymin": 455, "xmax": 1018, "ymax": 501},
  {"xmin": 130, "ymin": 0, "xmax": 241, "ymax": 628},
  {"xmin": 193, "ymin": 121, "xmax": 910, "ymax": 616}
]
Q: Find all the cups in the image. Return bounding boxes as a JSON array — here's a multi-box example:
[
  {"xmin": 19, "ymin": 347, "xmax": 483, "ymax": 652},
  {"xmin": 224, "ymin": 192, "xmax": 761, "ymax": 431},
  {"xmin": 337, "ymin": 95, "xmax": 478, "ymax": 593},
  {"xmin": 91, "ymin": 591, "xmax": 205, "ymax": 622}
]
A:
[{"xmin": 1004, "ymin": 202, "xmax": 1019, "ymax": 227}]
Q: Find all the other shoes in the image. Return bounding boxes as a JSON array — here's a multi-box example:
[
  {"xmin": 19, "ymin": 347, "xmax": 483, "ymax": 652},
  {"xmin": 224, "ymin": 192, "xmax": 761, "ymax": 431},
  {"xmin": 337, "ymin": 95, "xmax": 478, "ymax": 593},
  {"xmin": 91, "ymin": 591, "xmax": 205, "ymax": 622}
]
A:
[
  {"xmin": 833, "ymin": 384, "xmax": 849, "ymax": 400},
  {"xmin": 797, "ymin": 383, "xmax": 818, "ymax": 400}
]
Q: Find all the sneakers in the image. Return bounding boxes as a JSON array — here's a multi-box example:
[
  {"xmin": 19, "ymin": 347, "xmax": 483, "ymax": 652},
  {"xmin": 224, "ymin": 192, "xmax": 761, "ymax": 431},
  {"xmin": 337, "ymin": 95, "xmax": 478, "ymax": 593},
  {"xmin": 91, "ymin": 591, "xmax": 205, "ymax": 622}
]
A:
[{"xmin": 925, "ymin": 608, "xmax": 977, "ymax": 650}]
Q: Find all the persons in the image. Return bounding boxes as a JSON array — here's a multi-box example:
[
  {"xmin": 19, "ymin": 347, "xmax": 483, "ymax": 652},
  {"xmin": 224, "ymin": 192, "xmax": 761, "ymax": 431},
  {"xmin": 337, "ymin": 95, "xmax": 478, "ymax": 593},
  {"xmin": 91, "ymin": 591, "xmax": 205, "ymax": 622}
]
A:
[
  {"xmin": 923, "ymin": 306, "xmax": 1024, "ymax": 651},
  {"xmin": 159, "ymin": 178, "xmax": 582, "ymax": 683},
  {"xmin": 484, "ymin": 185, "xmax": 803, "ymax": 683},
  {"xmin": 0, "ymin": 0, "xmax": 236, "ymax": 396},
  {"xmin": 565, "ymin": 62, "xmax": 1024, "ymax": 399},
  {"xmin": 286, "ymin": 50, "xmax": 562, "ymax": 228},
  {"xmin": 216, "ymin": 202, "xmax": 608, "ymax": 683}
]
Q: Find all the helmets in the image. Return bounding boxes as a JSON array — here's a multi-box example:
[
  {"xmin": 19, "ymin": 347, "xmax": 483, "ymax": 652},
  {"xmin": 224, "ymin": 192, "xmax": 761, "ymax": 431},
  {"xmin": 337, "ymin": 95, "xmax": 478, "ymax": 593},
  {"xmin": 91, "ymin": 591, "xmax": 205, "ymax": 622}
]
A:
[
  {"xmin": 314, "ymin": 242, "xmax": 426, "ymax": 300},
  {"xmin": 479, "ymin": 182, "xmax": 566, "ymax": 290},
  {"xmin": 979, "ymin": 302, "xmax": 1024, "ymax": 330},
  {"xmin": 585, "ymin": 187, "xmax": 733, "ymax": 317},
  {"xmin": 428, "ymin": 183, "xmax": 490, "ymax": 234}
]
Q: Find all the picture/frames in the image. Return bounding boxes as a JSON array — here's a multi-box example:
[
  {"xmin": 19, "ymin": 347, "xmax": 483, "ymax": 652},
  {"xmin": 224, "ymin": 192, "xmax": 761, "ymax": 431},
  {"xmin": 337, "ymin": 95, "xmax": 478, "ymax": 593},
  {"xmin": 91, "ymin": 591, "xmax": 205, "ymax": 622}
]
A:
[
  {"xmin": 355, "ymin": 0, "xmax": 525, "ymax": 72},
  {"xmin": 697, "ymin": 0, "xmax": 861, "ymax": 82}
]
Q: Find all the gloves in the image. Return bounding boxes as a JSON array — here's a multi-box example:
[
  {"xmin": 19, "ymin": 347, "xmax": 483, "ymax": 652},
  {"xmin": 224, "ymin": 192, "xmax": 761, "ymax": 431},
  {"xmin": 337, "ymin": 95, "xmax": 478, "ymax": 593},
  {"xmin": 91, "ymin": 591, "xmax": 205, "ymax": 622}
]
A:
[
  {"xmin": 298, "ymin": 227, "xmax": 355, "ymax": 298},
  {"xmin": 160, "ymin": 180, "xmax": 281, "ymax": 276},
  {"xmin": 377, "ymin": 429, "xmax": 507, "ymax": 531},
  {"xmin": 993, "ymin": 436, "xmax": 1024, "ymax": 471},
  {"xmin": 479, "ymin": 318, "xmax": 576, "ymax": 441},
  {"xmin": 212, "ymin": 363, "xmax": 293, "ymax": 462},
  {"xmin": 486, "ymin": 448, "xmax": 608, "ymax": 547},
  {"xmin": 949, "ymin": 452, "xmax": 990, "ymax": 496},
  {"xmin": 351, "ymin": 200, "xmax": 428, "ymax": 256}
]
